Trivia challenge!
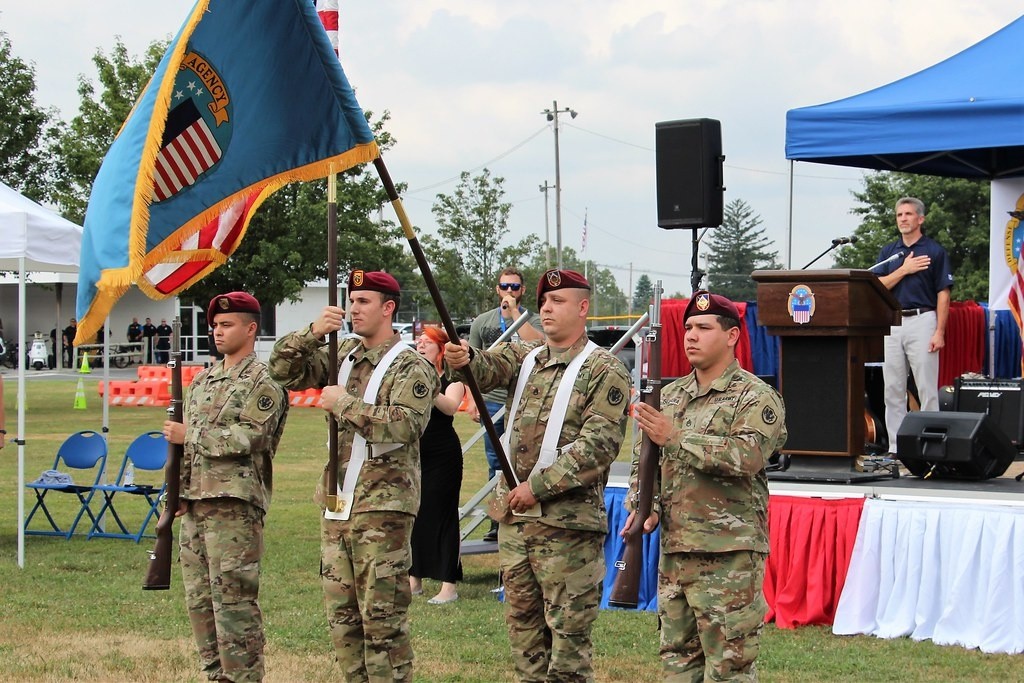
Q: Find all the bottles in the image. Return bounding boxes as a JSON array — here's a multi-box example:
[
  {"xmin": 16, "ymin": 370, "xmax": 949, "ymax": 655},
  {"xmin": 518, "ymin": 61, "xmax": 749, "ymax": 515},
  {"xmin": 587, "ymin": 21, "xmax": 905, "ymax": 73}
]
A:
[{"xmin": 123, "ymin": 463, "xmax": 134, "ymax": 487}]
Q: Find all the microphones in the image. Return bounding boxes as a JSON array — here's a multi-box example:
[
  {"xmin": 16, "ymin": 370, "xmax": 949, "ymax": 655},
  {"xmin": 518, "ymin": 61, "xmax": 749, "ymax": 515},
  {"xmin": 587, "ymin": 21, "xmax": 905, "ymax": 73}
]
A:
[
  {"xmin": 868, "ymin": 251, "xmax": 904, "ymax": 271},
  {"xmin": 832, "ymin": 236, "xmax": 858, "ymax": 244},
  {"xmin": 503, "ymin": 301, "xmax": 508, "ymax": 309}
]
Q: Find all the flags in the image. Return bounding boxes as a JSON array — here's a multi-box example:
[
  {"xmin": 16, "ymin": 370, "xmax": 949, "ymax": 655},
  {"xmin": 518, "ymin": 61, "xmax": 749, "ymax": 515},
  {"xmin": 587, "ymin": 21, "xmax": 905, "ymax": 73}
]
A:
[
  {"xmin": 1008, "ymin": 241, "xmax": 1024, "ymax": 381},
  {"xmin": 71, "ymin": 0, "xmax": 381, "ymax": 351}
]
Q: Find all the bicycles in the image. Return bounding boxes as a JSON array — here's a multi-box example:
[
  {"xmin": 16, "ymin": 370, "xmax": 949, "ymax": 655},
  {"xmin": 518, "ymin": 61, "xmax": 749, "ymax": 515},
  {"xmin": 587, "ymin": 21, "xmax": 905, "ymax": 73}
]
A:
[
  {"xmin": 2, "ymin": 338, "xmax": 17, "ymax": 370},
  {"xmin": 92, "ymin": 345, "xmax": 130, "ymax": 369}
]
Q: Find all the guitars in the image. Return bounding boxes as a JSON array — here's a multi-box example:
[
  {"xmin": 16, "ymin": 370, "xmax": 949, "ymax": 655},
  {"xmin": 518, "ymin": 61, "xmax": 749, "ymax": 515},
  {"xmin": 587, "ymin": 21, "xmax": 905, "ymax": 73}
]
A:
[{"xmin": 862, "ymin": 390, "xmax": 878, "ymax": 445}]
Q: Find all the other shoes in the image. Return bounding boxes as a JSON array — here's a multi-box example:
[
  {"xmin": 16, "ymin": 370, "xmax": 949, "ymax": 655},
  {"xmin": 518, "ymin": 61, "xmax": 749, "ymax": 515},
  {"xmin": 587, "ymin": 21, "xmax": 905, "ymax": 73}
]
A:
[
  {"xmin": 483, "ymin": 521, "xmax": 499, "ymax": 541},
  {"xmin": 411, "ymin": 588, "xmax": 423, "ymax": 594},
  {"xmin": 428, "ymin": 592, "xmax": 459, "ymax": 604}
]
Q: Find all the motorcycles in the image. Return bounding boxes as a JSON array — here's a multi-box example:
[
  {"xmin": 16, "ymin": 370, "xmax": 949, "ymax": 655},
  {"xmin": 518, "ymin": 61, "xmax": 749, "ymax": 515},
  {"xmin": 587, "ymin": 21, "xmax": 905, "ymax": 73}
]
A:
[{"xmin": 25, "ymin": 331, "xmax": 54, "ymax": 370}]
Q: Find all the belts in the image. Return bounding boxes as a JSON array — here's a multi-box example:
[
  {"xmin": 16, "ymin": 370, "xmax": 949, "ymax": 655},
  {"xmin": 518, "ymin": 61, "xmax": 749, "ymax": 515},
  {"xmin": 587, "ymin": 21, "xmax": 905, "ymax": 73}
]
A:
[{"xmin": 902, "ymin": 308, "xmax": 932, "ymax": 317}]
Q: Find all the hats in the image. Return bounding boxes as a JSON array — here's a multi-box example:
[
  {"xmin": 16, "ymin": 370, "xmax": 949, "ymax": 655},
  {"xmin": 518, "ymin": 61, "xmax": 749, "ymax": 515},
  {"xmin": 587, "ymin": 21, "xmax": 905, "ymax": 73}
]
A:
[
  {"xmin": 538, "ymin": 268, "xmax": 592, "ymax": 314},
  {"xmin": 208, "ymin": 292, "xmax": 261, "ymax": 328},
  {"xmin": 683, "ymin": 290, "xmax": 741, "ymax": 325},
  {"xmin": 349, "ymin": 269, "xmax": 401, "ymax": 297}
]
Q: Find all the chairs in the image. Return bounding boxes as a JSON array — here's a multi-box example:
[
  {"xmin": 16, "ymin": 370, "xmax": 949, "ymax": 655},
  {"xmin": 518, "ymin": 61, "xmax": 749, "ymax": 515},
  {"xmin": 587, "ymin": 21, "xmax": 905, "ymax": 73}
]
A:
[
  {"xmin": 86, "ymin": 431, "xmax": 168, "ymax": 544},
  {"xmin": 24, "ymin": 431, "xmax": 108, "ymax": 540}
]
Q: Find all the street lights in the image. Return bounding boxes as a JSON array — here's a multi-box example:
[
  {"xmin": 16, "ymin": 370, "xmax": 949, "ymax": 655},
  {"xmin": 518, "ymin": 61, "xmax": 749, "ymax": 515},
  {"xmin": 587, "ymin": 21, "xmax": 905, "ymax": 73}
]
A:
[
  {"xmin": 539, "ymin": 180, "xmax": 556, "ymax": 269},
  {"xmin": 540, "ymin": 100, "xmax": 578, "ymax": 268}
]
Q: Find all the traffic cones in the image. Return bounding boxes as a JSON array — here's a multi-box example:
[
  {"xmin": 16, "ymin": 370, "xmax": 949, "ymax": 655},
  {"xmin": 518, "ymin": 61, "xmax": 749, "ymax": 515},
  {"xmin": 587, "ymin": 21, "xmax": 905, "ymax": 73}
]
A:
[
  {"xmin": 73, "ymin": 377, "xmax": 87, "ymax": 410},
  {"xmin": 79, "ymin": 352, "xmax": 91, "ymax": 373}
]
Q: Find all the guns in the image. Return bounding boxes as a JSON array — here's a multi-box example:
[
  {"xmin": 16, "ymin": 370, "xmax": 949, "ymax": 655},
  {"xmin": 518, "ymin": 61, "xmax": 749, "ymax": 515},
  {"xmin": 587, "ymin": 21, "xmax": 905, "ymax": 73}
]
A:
[
  {"xmin": 140, "ymin": 315, "xmax": 184, "ymax": 592},
  {"xmin": 608, "ymin": 277, "xmax": 666, "ymax": 610}
]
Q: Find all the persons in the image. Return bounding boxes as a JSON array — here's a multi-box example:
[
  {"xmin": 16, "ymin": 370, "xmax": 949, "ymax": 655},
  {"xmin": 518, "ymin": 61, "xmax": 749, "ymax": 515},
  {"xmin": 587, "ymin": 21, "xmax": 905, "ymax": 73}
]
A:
[
  {"xmin": 157, "ymin": 291, "xmax": 289, "ymax": 683},
  {"xmin": 464, "ymin": 268, "xmax": 548, "ymax": 541},
  {"xmin": 267, "ymin": 270, "xmax": 437, "ymax": 683},
  {"xmin": 444, "ymin": 268, "xmax": 634, "ymax": 682},
  {"xmin": 0, "ymin": 373, "xmax": 5, "ymax": 450},
  {"xmin": 48, "ymin": 318, "xmax": 112, "ymax": 368},
  {"xmin": 127, "ymin": 317, "xmax": 173, "ymax": 364},
  {"xmin": 619, "ymin": 289, "xmax": 788, "ymax": 683},
  {"xmin": 872, "ymin": 196, "xmax": 955, "ymax": 461},
  {"xmin": 407, "ymin": 325, "xmax": 465, "ymax": 605}
]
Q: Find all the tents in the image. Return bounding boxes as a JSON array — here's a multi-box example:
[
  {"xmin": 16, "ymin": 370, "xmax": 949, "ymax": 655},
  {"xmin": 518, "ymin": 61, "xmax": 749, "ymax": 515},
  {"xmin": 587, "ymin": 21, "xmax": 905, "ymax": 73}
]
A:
[
  {"xmin": 786, "ymin": 15, "xmax": 1023, "ymax": 270},
  {"xmin": 0, "ymin": 179, "xmax": 110, "ymax": 569}
]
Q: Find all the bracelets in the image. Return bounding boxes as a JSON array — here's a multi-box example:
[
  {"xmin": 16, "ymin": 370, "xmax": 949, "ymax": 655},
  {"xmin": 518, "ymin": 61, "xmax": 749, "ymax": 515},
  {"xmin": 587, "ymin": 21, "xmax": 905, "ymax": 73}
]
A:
[{"xmin": 0, "ymin": 430, "xmax": 6, "ymax": 434}]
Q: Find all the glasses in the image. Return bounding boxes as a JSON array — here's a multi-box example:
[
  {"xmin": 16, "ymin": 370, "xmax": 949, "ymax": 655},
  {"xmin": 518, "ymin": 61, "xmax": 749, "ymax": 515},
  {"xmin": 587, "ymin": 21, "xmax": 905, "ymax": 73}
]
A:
[
  {"xmin": 415, "ymin": 339, "xmax": 433, "ymax": 346},
  {"xmin": 498, "ymin": 283, "xmax": 522, "ymax": 291}
]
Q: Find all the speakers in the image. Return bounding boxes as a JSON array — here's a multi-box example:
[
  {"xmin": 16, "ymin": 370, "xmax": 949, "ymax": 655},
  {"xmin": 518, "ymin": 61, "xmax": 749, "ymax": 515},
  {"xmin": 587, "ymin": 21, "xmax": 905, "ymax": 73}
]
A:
[
  {"xmin": 655, "ymin": 118, "xmax": 724, "ymax": 230},
  {"xmin": 896, "ymin": 377, "xmax": 1024, "ymax": 481}
]
